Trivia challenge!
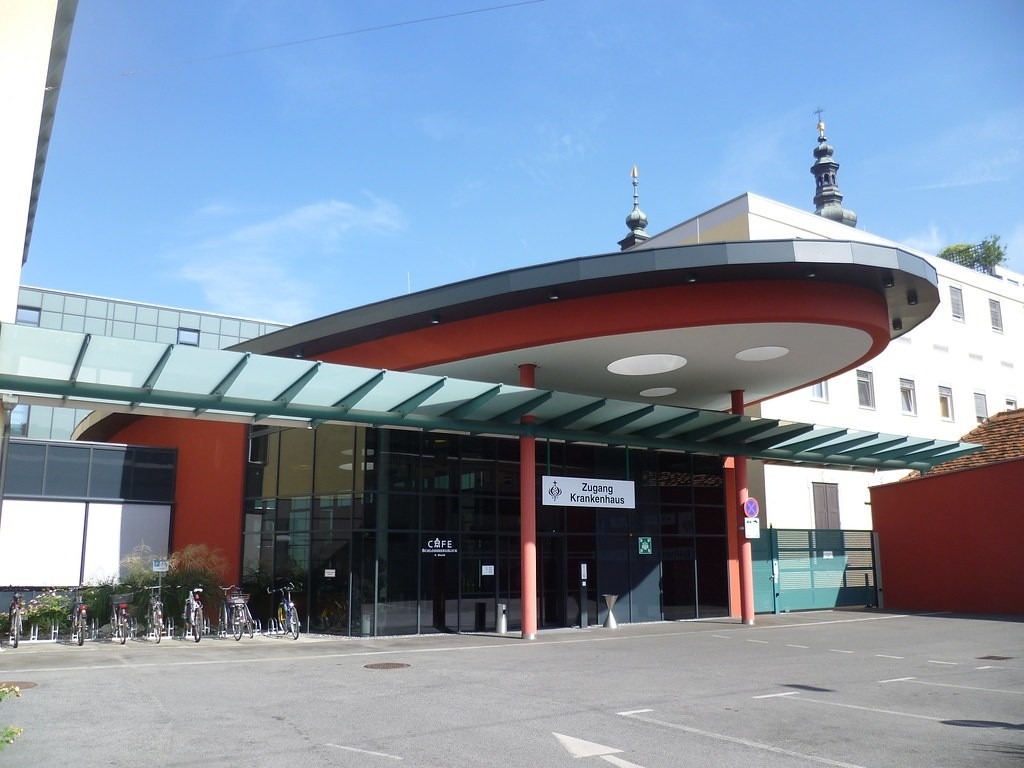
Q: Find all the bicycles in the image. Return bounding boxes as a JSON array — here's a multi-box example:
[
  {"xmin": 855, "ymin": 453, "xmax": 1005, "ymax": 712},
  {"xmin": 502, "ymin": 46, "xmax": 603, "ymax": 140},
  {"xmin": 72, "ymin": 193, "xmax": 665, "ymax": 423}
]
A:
[
  {"xmin": 3, "ymin": 588, "xmax": 29, "ymax": 648},
  {"xmin": 213, "ymin": 584, "xmax": 255, "ymax": 641},
  {"xmin": 98, "ymin": 582, "xmax": 130, "ymax": 645},
  {"xmin": 266, "ymin": 582, "xmax": 301, "ymax": 640},
  {"xmin": 67, "ymin": 585, "xmax": 89, "ymax": 646},
  {"xmin": 138, "ymin": 584, "xmax": 171, "ymax": 643},
  {"xmin": 175, "ymin": 583, "xmax": 204, "ymax": 642}
]
